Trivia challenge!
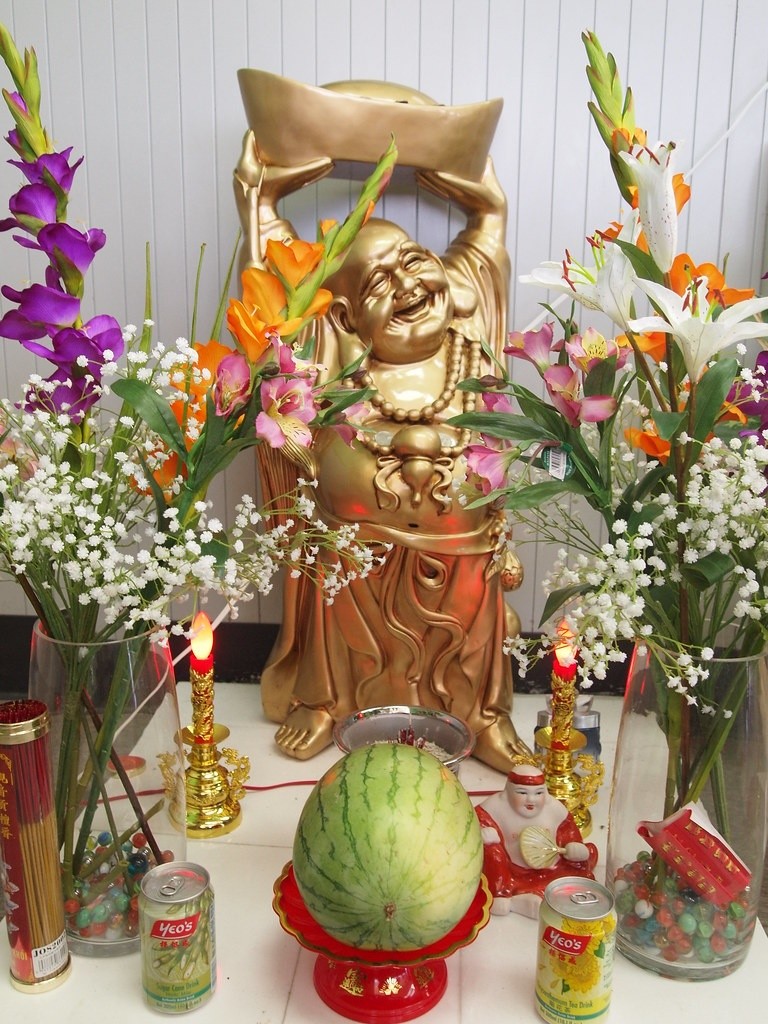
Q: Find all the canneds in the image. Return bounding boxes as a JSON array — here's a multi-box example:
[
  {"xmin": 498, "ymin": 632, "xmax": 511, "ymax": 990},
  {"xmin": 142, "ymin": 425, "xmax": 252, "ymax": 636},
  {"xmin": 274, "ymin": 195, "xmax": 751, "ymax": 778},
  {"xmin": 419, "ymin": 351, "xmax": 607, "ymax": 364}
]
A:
[
  {"xmin": 534, "ymin": 876, "xmax": 618, "ymax": 1024},
  {"xmin": 137, "ymin": 861, "xmax": 218, "ymax": 1015}
]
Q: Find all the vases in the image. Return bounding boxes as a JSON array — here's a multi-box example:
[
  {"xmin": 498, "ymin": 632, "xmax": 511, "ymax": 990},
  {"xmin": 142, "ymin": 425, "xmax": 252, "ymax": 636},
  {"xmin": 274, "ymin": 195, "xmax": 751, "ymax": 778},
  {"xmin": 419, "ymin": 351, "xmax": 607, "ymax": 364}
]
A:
[
  {"xmin": 28, "ymin": 617, "xmax": 188, "ymax": 959},
  {"xmin": 603, "ymin": 618, "xmax": 768, "ymax": 981}
]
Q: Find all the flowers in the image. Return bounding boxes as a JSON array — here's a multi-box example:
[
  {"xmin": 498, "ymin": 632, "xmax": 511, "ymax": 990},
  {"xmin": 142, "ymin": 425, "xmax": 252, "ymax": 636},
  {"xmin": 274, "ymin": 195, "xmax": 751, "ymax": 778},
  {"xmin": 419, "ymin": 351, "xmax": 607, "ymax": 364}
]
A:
[
  {"xmin": 0, "ymin": 23, "xmax": 399, "ymax": 896},
  {"xmin": 438, "ymin": 29, "xmax": 768, "ymax": 921}
]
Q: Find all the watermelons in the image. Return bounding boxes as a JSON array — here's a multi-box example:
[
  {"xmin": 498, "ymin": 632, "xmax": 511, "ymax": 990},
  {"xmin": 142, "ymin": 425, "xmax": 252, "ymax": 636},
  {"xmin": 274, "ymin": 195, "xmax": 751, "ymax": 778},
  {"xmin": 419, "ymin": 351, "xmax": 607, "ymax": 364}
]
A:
[{"xmin": 292, "ymin": 741, "xmax": 483, "ymax": 952}]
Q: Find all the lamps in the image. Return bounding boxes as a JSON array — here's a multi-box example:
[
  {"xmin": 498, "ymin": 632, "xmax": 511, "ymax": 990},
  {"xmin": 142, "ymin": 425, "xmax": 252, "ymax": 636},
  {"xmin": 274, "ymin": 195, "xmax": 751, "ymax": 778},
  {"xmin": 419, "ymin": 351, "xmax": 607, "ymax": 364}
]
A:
[
  {"xmin": 511, "ymin": 619, "xmax": 606, "ymax": 840},
  {"xmin": 151, "ymin": 612, "xmax": 251, "ymax": 841}
]
